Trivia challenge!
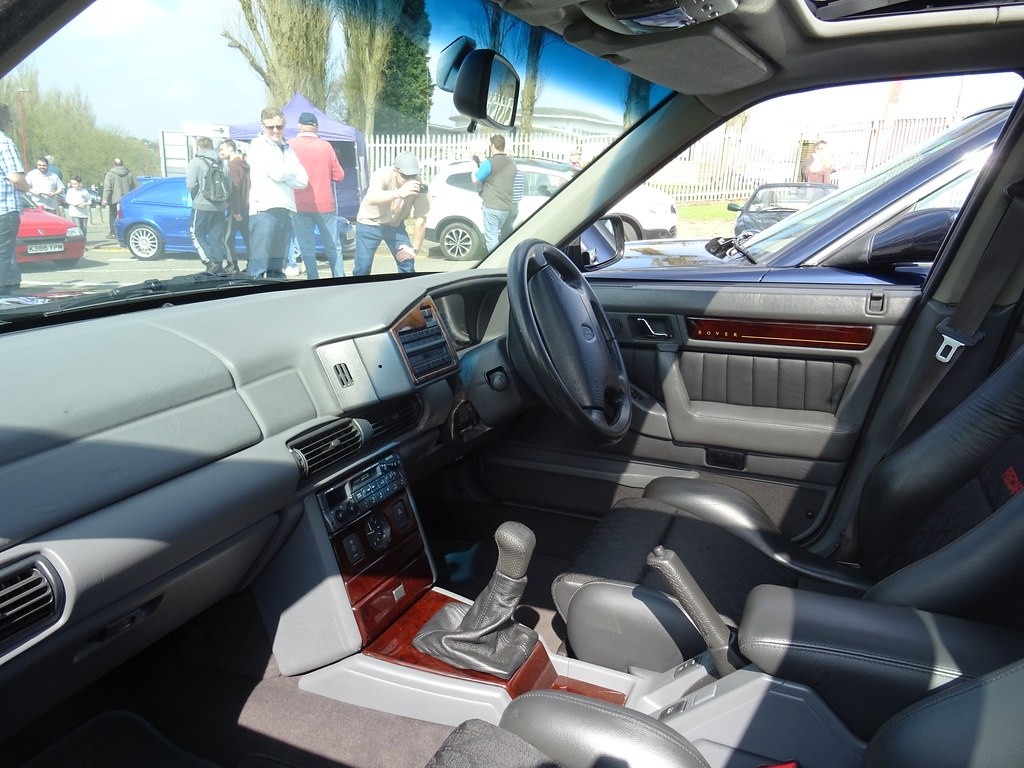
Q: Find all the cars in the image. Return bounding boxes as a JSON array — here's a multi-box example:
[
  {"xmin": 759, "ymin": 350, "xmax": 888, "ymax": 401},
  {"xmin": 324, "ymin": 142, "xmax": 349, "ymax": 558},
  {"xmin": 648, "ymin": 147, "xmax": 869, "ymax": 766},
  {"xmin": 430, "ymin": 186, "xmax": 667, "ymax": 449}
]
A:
[
  {"xmin": 727, "ymin": 183, "xmax": 839, "ymax": 237},
  {"xmin": 85, "ymin": 189, "xmax": 103, "ymax": 208},
  {"xmin": 113, "ymin": 175, "xmax": 355, "ymax": 262},
  {"xmin": 14, "ymin": 191, "xmax": 87, "ymax": 267},
  {"xmin": 597, "ymin": 99, "xmax": 1017, "ymax": 271}
]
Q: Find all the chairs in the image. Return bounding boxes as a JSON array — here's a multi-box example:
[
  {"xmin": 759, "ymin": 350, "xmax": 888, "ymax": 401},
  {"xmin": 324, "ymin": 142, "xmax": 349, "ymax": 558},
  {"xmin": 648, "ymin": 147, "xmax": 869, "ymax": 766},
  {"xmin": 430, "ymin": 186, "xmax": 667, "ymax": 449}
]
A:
[
  {"xmin": 552, "ymin": 343, "xmax": 1024, "ymax": 673},
  {"xmin": 426, "ymin": 657, "xmax": 1023, "ymax": 768}
]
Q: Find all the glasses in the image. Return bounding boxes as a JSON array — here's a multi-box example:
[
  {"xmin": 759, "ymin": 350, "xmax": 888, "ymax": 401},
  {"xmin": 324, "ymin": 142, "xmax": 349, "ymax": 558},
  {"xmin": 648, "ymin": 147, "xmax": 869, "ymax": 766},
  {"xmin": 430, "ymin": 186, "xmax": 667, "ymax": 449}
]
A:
[
  {"xmin": 262, "ymin": 121, "xmax": 284, "ymax": 130},
  {"xmin": 398, "ymin": 172, "xmax": 417, "ymax": 179}
]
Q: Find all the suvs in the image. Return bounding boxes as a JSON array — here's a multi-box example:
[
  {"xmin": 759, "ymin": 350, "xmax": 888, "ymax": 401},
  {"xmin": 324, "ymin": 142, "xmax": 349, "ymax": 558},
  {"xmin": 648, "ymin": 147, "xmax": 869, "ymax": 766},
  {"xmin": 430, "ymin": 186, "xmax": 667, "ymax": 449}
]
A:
[{"xmin": 422, "ymin": 155, "xmax": 678, "ymax": 261}]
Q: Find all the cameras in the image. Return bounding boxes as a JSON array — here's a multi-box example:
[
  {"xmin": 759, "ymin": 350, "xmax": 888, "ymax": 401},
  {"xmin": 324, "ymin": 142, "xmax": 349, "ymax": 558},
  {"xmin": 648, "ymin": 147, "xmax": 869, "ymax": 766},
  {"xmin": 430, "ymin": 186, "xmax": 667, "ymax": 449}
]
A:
[{"xmin": 417, "ymin": 185, "xmax": 428, "ymax": 194}]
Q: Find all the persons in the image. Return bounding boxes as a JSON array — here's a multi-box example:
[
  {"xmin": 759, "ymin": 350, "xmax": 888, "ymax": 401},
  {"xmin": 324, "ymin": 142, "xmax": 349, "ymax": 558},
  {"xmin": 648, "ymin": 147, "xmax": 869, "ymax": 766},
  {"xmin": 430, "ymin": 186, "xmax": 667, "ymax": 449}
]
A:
[
  {"xmin": 353, "ymin": 150, "xmax": 430, "ymax": 275},
  {"xmin": 471, "ymin": 135, "xmax": 517, "ymax": 253},
  {"xmin": 186, "ymin": 137, "xmax": 250, "ymax": 273},
  {"xmin": 66, "ymin": 176, "xmax": 93, "ymax": 236},
  {"xmin": 102, "ymin": 157, "xmax": 132, "ymax": 240},
  {"xmin": 245, "ymin": 108, "xmax": 310, "ymax": 278},
  {"xmin": 802, "ymin": 140, "xmax": 836, "ymax": 182},
  {"xmin": 559, "ymin": 149, "xmax": 582, "ymax": 185},
  {"xmin": 503, "ymin": 171, "xmax": 524, "ymax": 241},
  {"xmin": 0, "ymin": 102, "xmax": 30, "ymax": 288},
  {"xmin": 25, "ymin": 155, "xmax": 65, "ymax": 215},
  {"xmin": 285, "ymin": 112, "xmax": 346, "ymax": 279}
]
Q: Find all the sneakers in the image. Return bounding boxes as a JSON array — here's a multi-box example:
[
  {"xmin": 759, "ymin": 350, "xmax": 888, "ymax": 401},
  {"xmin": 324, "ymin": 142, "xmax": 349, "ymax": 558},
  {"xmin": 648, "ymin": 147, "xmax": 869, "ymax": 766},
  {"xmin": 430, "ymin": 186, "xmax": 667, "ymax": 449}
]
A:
[
  {"xmin": 284, "ymin": 267, "xmax": 299, "ymax": 276},
  {"xmin": 106, "ymin": 234, "xmax": 116, "ymax": 239}
]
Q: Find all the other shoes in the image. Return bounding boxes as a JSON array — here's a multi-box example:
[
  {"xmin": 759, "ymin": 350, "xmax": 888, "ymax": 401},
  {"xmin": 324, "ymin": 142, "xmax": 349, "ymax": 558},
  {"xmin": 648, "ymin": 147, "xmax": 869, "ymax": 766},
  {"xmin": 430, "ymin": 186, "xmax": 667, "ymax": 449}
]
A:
[{"xmin": 206, "ymin": 262, "xmax": 220, "ymax": 274}]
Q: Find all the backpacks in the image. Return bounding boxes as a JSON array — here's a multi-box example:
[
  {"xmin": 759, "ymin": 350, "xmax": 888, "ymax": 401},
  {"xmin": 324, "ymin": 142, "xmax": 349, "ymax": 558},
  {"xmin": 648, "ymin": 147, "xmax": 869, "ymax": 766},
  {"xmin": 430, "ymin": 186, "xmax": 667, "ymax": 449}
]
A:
[{"xmin": 199, "ymin": 156, "xmax": 229, "ymax": 202}]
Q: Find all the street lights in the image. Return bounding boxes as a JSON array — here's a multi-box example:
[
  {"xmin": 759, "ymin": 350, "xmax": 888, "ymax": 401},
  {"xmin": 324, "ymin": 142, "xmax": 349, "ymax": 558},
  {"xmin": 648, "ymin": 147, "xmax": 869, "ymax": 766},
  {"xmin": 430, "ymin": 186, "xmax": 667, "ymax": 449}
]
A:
[{"xmin": 18, "ymin": 87, "xmax": 34, "ymax": 172}]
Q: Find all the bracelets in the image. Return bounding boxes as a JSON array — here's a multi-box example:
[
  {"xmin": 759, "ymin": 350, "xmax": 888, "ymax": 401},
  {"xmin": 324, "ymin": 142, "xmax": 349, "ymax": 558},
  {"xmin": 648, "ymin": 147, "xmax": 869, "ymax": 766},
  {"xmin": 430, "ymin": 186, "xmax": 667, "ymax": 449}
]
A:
[{"xmin": 413, "ymin": 248, "xmax": 419, "ymax": 254}]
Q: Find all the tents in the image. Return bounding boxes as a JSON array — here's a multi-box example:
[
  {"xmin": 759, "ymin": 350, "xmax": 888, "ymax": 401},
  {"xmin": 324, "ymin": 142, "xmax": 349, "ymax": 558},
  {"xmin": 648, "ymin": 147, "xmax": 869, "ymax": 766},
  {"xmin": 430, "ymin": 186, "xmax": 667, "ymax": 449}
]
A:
[{"xmin": 228, "ymin": 91, "xmax": 370, "ymax": 223}]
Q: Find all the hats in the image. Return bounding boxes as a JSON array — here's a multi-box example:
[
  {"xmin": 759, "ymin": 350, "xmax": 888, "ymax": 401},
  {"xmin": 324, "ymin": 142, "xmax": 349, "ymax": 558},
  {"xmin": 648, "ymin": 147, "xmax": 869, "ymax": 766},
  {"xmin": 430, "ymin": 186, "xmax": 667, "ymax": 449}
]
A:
[
  {"xmin": 298, "ymin": 112, "xmax": 319, "ymax": 126},
  {"xmin": 396, "ymin": 151, "xmax": 420, "ymax": 175}
]
